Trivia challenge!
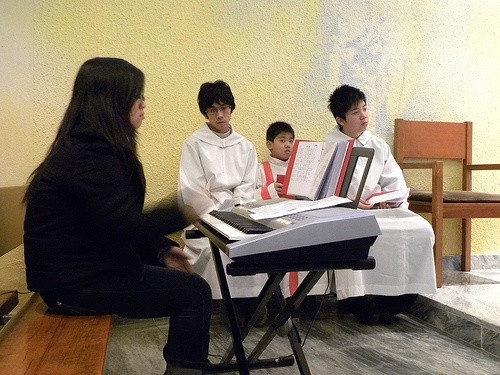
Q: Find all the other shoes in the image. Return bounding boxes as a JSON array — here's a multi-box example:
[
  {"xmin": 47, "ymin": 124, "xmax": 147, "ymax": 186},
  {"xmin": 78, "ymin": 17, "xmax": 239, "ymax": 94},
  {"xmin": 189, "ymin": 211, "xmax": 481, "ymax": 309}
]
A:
[
  {"xmin": 372, "ymin": 299, "xmax": 394, "ymax": 325},
  {"xmin": 354, "ymin": 299, "xmax": 379, "ymax": 325},
  {"xmin": 254, "ymin": 307, "xmax": 268, "ymax": 327}
]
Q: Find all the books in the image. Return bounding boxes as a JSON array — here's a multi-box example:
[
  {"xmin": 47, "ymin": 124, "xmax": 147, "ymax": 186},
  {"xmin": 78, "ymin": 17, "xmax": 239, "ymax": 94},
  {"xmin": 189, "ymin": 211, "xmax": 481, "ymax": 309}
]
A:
[
  {"xmin": 277, "ymin": 175, "xmax": 292, "ymax": 199},
  {"xmin": 280, "ymin": 139, "xmax": 353, "ymax": 202},
  {"xmin": 363, "ymin": 188, "xmax": 407, "ymax": 209}
]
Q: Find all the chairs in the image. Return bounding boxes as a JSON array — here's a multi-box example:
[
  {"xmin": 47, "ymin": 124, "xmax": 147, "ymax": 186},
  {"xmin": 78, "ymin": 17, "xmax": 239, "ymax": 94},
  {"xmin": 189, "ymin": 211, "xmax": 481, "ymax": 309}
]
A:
[{"xmin": 395, "ymin": 118, "xmax": 500, "ymax": 289}]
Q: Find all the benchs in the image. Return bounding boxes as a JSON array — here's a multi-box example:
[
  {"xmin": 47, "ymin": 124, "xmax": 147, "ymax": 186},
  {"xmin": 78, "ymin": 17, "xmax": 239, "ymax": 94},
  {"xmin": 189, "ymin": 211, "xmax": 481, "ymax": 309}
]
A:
[{"xmin": 0, "ymin": 183, "xmax": 114, "ymax": 375}]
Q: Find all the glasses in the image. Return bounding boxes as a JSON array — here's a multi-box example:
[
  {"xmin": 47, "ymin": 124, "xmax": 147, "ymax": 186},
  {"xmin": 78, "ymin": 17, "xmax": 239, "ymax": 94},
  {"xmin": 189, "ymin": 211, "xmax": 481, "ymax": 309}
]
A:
[{"xmin": 204, "ymin": 103, "xmax": 233, "ymax": 118}]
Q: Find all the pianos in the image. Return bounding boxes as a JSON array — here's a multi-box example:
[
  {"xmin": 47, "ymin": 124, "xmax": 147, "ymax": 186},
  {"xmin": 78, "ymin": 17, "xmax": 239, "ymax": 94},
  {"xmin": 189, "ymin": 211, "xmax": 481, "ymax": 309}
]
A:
[{"xmin": 186, "ymin": 194, "xmax": 381, "ymax": 375}]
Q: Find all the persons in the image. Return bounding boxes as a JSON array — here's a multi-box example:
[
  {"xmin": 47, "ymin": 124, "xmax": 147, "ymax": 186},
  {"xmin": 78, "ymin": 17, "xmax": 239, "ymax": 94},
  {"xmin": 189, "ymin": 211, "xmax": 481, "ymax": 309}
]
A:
[
  {"xmin": 256, "ymin": 122, "xmax": 322, "ymax": 322},
  {"xmin": 20, "ymin": 57, "xmax": 213, "ymax": 375},
  {"xmin": 179, "ymin": 79, "xmax": 266, "ymax": 329},
  {"xmin": 326, "ymin": 84, "xmax": 437, "ymax": 325}
]
